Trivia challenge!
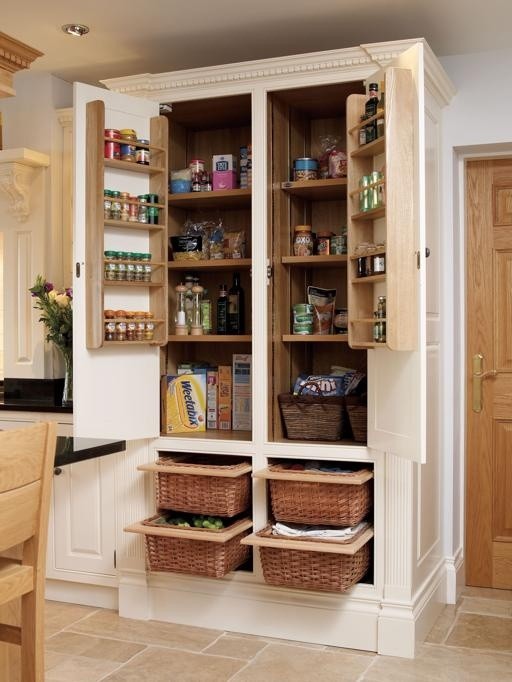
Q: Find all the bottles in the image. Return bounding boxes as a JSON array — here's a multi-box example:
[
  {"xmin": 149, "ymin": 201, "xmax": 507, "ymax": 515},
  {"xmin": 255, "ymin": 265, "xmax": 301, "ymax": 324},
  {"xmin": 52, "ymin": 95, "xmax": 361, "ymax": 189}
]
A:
[
  {"xmin": 173, "ymin": 272, "xmax": 245, "ymax": 334},
  {"xmin": 360, "ymin": 80, "xmax": 385, "ymax": 145},
  {"xmin": 373, "ymin": 297, "xmax": 386, "ymax": 344}
]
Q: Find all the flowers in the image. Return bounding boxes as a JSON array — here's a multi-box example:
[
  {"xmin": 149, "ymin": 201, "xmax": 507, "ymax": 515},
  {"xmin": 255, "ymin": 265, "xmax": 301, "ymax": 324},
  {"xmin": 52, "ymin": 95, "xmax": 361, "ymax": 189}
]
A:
[{"xmin": 28, "ymin": 273, "xmax": 73, "ymax": 379}]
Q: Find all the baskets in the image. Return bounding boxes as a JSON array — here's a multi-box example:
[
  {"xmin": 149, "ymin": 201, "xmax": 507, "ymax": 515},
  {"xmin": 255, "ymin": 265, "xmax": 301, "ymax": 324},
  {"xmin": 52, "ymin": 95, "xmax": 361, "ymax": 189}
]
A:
[
  {"xmin": 123, "ymin": 458, "xmax": 375, "ymax": 595},
  {"xmin": 278, "ymin": 394, "xmax": 367, "ymax": 440}
]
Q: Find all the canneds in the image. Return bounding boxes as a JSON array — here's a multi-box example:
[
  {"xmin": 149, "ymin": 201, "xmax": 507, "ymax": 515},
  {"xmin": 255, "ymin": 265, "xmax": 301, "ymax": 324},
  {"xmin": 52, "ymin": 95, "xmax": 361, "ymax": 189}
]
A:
[
  {"xmin": 358, "ymin": 171, "xmax": 382, "ymax": 212},
  {"xmin": 293, "ymin": 303, "xmax": 314, "ymax": 335},
  {"xmin": 294, "ymin": 157, "xmax": 319, "ymax": 180},
  {"xmin": 105, "ymin": 128, "xmax": 150, "ymax": 165}
]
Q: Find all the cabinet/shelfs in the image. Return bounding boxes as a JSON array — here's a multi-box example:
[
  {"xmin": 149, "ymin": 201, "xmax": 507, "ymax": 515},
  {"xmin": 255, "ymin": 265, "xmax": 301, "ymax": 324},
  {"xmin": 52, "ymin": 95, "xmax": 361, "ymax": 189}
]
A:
[
  {"xmin": 72, "ymin": 38, "xmax": 458, "ymax": 661},
  {"xmin": 0, "ymin": 411, "xmax": 118, "ymax": 610}
]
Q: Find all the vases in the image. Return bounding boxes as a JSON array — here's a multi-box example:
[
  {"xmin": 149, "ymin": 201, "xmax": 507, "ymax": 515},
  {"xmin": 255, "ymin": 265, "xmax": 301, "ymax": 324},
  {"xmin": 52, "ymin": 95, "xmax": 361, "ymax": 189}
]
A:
[{"xmin": 62, "ymin": 348, "xmax": 72, "ymax": 405}]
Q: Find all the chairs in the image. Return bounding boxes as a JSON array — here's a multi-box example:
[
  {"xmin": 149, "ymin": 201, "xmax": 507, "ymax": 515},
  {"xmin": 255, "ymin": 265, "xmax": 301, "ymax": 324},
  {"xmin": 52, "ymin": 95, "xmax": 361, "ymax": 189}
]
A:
[{"xmin": 0, "ymin": 420, "xmax": 58, "ymax": 682}]
